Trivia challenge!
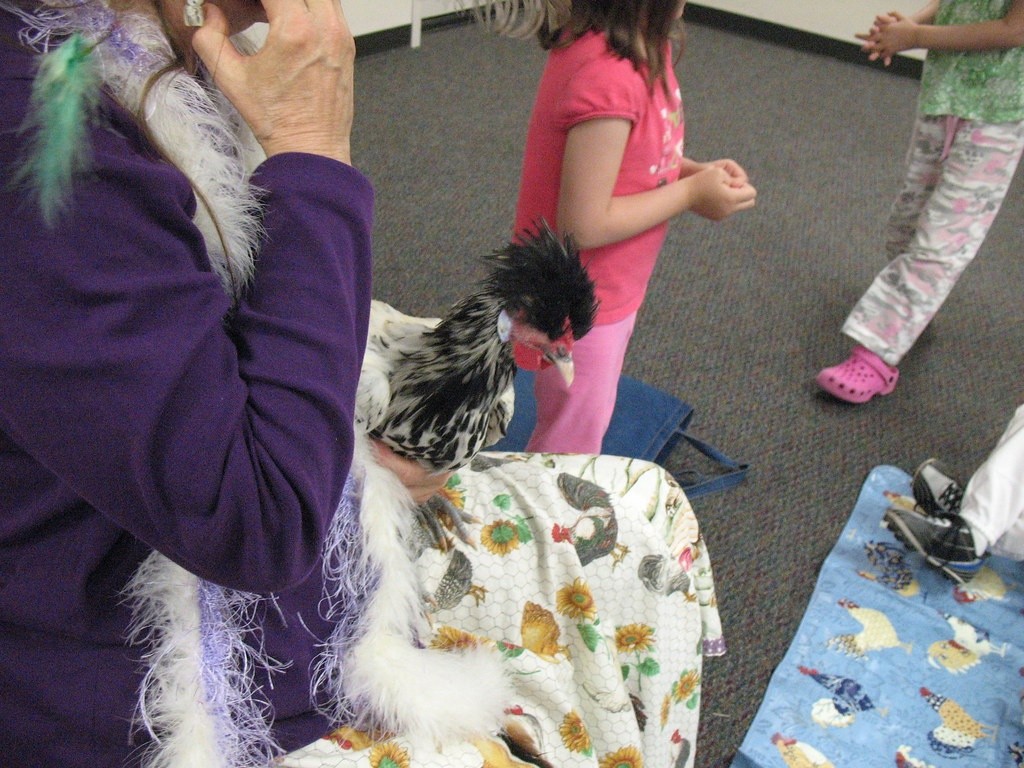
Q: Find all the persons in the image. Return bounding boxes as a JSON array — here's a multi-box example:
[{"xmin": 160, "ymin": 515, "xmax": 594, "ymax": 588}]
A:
[
  {"xmin": 815, "ymin": 0, "xmax": 1024, "ymax": 402},
  {"xmin": 883, "ymin": 401, "xmax": 1024, "ymax": 580},
  {"xmin": 0, "ymin": 0, "xmax": 729, "ymax": 768},
  {"xmin": 510, "ymin": 1, "xmax": 758, "ymax": 455}
]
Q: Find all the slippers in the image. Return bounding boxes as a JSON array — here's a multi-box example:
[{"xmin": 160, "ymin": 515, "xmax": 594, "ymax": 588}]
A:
[{"xmin": 816, "ymin": 344, "xmax": 899, "ymax": 404}]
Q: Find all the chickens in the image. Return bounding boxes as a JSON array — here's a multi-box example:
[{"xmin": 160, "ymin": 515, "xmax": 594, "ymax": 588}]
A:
[{"xmin": 336, "ymin": 211, "xmax": 607, "ymax": 553}]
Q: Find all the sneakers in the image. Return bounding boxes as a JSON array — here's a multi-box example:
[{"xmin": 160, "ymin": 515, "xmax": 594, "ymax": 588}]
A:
[
  {"xmin": 910, "ymin": 457, "xmax": 964, "ymax": 517},
  {"xmin": 884, "ymin": 509, "xmax": 992, "ymax": 586}
]
